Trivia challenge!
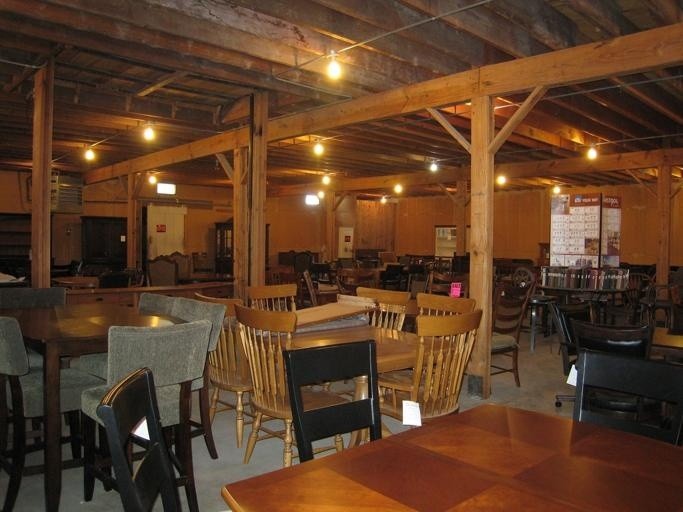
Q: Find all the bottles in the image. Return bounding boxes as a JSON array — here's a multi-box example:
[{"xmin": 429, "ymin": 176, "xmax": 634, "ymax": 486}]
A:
[{"xmin": 542, "ymin": 268, "xmax": 547, "ymax": 287}]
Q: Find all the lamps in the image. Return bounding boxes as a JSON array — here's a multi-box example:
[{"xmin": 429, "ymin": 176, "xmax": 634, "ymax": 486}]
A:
[
  {"xmin": 327, "ymin": 56, "xmax": 341, "ymax": 79},
  {"xmin": 430, "ymin": 159, "xmax": 437, "ymax": 172}
]
